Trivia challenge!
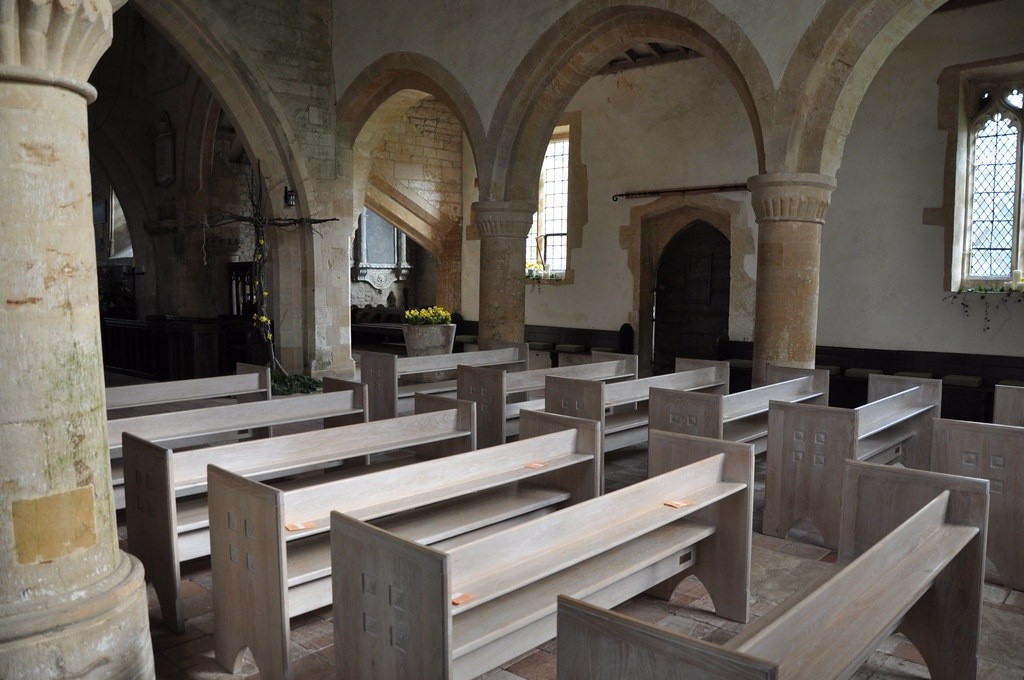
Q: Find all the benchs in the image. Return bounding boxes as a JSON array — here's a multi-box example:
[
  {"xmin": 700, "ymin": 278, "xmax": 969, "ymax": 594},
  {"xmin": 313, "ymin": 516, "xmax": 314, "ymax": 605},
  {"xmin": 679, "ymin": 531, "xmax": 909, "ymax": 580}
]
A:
[
  {"xmin": 351, "ymin": 304, "xmax": 404, "ymax": 342},
  {"xmin": 105, "ymin": 338, "xmax": 1024, "ymax": 680}
]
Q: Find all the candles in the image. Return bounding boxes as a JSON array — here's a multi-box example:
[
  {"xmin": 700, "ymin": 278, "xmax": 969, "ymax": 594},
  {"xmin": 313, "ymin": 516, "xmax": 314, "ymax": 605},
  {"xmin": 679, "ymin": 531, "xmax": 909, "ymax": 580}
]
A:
[
  {"xmin": 132, "ymin": 258, "xmax": 135, "ymax": 267},
  {"xmin": 1003, "ymin": 270, "xmax": 1024, "ymax": 292},
  {"xmin": 142, "ymin": 265, "xmax": 145, "ymax": 272},
  {"xmin": 123, "ymin": 266, "xmax": 126, "ymax": 272}
]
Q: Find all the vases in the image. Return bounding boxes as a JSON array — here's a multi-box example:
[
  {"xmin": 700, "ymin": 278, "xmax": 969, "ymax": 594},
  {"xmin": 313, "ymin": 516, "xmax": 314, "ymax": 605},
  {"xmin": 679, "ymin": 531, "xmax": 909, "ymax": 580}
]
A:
[{"xmin": 402, "ymin": 323, "xmax": 456, "ymax": 357}]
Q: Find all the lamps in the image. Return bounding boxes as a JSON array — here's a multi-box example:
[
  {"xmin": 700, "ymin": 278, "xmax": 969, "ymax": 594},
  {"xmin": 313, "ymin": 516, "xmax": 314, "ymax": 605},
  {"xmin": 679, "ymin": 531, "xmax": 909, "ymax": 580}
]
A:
[{"xmin": 284, "ymin": 186, "xmax": 296, "ymax": 206}]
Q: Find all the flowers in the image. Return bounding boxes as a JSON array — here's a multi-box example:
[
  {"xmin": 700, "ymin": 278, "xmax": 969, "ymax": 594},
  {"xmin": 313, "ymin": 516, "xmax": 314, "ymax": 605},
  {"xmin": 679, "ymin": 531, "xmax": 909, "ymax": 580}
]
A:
[
  {"xmin": 404, "ymin": 305, "xmax": 452, "ymax": 324},
  {"xmin": 253, "ymin": 240, "xmax": 324, "ymax": 394},
  {"xmin": 525, "ymin": 262, "xmax": 542, "ymax": 293}
]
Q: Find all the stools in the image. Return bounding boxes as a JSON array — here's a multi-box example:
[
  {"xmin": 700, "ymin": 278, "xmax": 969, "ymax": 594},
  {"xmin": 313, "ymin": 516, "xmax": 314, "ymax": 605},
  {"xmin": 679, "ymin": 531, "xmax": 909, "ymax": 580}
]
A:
[
  {"xmin": 725, "ymin": 359, "xmax": 1024, "ymax": 422},
  {"xmin": 455, "ymin": 335, "xmax": 618, "ymax": 370}
]
